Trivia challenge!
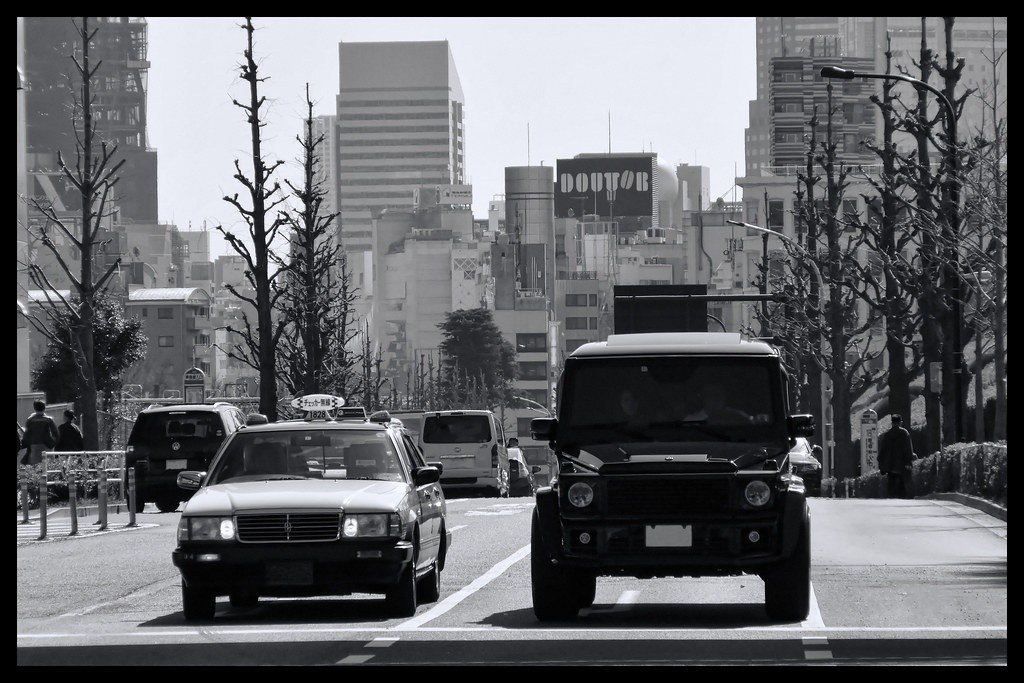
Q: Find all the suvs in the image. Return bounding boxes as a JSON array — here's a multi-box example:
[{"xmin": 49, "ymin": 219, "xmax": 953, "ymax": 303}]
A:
[{"xmin": 529, "ymin": 331, "xmax": 820, "ymax": 624}]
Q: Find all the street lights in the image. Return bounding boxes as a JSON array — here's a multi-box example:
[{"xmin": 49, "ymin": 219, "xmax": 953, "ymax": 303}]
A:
[
  {"xmin": 513, "ymin": 395, "xmax": 552, "ymax": 418},
  {"xmin": 816, "ymin": 65, "xmax": 963, "ymax": 441},
  {"xmin": 725, "ymin": 219, "xmax": 827, "ymax": 440}
]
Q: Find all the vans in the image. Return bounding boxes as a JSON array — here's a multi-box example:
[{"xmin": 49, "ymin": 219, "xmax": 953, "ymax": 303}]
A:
[{"xmin": 417, "ymin": 402, "xmax": 510, "ymax": 499}]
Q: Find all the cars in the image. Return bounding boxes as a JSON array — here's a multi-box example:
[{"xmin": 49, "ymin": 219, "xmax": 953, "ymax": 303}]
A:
[
  {"xmin": 170, "ymin": 414, "xmax": 453, "ymax": 618},
  {"xmin": 788, "ymin": 430, "xmax": 826, "ymax": 497},
  {"xmin": 121, "ymin": 397, "xmax": 246, "ymax": 510}
]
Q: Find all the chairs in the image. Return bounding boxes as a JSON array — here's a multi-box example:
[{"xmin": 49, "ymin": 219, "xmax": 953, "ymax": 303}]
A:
[
  {"xmin": 346, "ymin": 443, "xmax": 388, "ymax": 479},
  {"xmin": 284, "ymin": 445, "xmax": 310, "ymax": 473},
  {"xmin": 182, "ymin": 423, "xmax": 196, "ymax": 437},
  {"xmin": 167, "ymin": 421, "xmax": 180, "ymax": 436},
  {"xmin": 244, "ymin": 442, "xmax": 287, "ymax": 476}
]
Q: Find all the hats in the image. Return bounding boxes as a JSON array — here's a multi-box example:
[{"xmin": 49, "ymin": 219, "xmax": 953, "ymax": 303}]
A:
[
  {"xmin": 890, "ymin": 414, "xmax": 902, "ymax": 424},
  {"xmin": 33, "ymin": 400, "xmax": 45, "ymax": 412},
  {"xmin": 64, "ymin": 410, "xmax": 76, "ymax": 421}
]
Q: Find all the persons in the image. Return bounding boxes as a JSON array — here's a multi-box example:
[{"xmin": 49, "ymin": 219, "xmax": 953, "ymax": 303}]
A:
[
  {"xmin": 20, "ymin": 400, "xmax": 83, "ymax": 464},
  {"xmin": 877, "ymin": 413, "xmax": 913, "ymax": 499},
  {"xmin": 681, "ymin": 377, "xmax": 734, "ymax": 427}
]
